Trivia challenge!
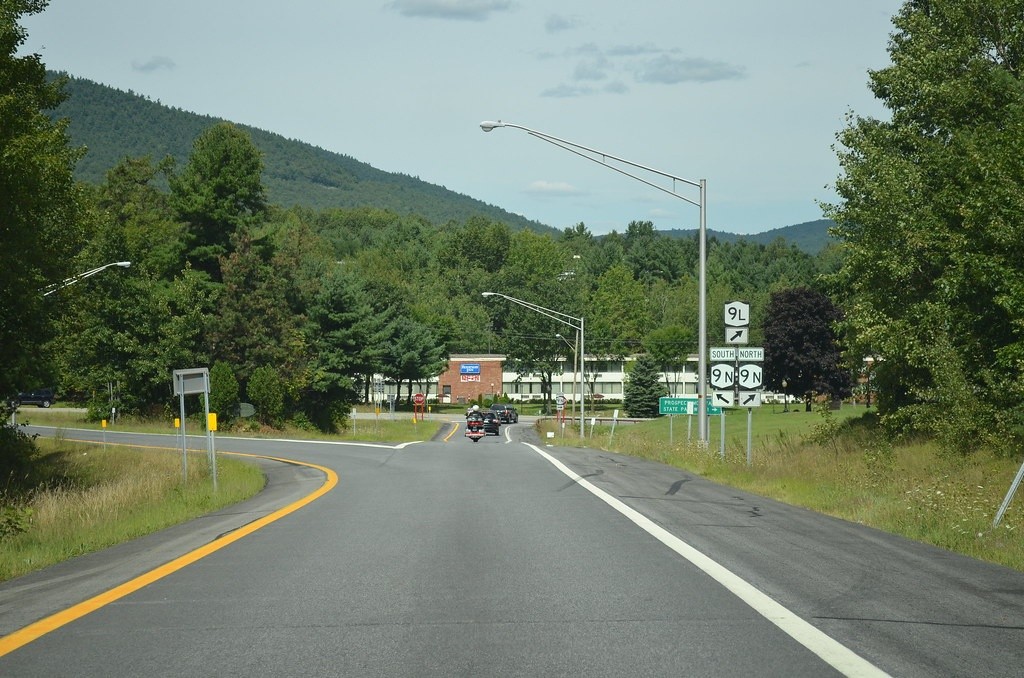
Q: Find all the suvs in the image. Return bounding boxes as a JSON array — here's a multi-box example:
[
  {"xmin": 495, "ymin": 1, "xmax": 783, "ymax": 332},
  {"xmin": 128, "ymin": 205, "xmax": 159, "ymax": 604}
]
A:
[{"xmin": 6, "ymin": 389, "xmax": 56, "ymax": 408}]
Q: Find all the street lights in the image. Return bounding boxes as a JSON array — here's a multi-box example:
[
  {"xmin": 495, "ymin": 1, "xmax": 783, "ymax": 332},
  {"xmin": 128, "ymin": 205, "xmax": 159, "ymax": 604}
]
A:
[
  {"xmin": 482, "ymin": 292, "xmax": 586, "ymax": 437},
  {"xmin": 478, "ymin": 119, "xmax": 709, "ymax": 449},
  {"xmin": 36, "ymin": 261, "xmax": 132, "ymax": 298}
]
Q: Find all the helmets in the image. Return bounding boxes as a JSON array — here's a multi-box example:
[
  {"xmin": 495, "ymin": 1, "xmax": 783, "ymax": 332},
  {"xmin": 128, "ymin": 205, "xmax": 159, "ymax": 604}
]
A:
[{"xmin": 473, "ymin": 405, "xmax": 480, "ymax": 412}]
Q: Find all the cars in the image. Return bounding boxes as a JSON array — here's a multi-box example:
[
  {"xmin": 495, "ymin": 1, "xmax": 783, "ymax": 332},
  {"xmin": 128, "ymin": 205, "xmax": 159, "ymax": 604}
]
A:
[
  {"xmin": 465, "ymin": 411, "xmax": 499, "ymax": 436},
  {"xmin": 488, "ymin": 404, "xmax": 518, "ymax": 424}
]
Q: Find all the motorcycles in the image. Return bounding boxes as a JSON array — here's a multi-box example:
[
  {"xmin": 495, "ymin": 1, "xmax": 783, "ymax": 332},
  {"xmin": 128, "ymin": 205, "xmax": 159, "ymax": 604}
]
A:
[{"xmin": 465, "ymin": 418, "xmax": 485, "ymax": 443}]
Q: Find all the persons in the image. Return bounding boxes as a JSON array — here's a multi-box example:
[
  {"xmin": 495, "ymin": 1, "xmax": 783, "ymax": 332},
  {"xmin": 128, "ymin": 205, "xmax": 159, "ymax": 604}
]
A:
[{"xmin": 466, "ymin": 404, "xmax": 486, "ymax": 421}]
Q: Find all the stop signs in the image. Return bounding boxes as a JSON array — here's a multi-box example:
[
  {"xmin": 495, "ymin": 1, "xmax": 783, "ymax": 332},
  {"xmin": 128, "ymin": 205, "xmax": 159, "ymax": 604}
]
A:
[{"xmin": 413, "ymin": 393, "xmax": 426, "ymax": 405}]
